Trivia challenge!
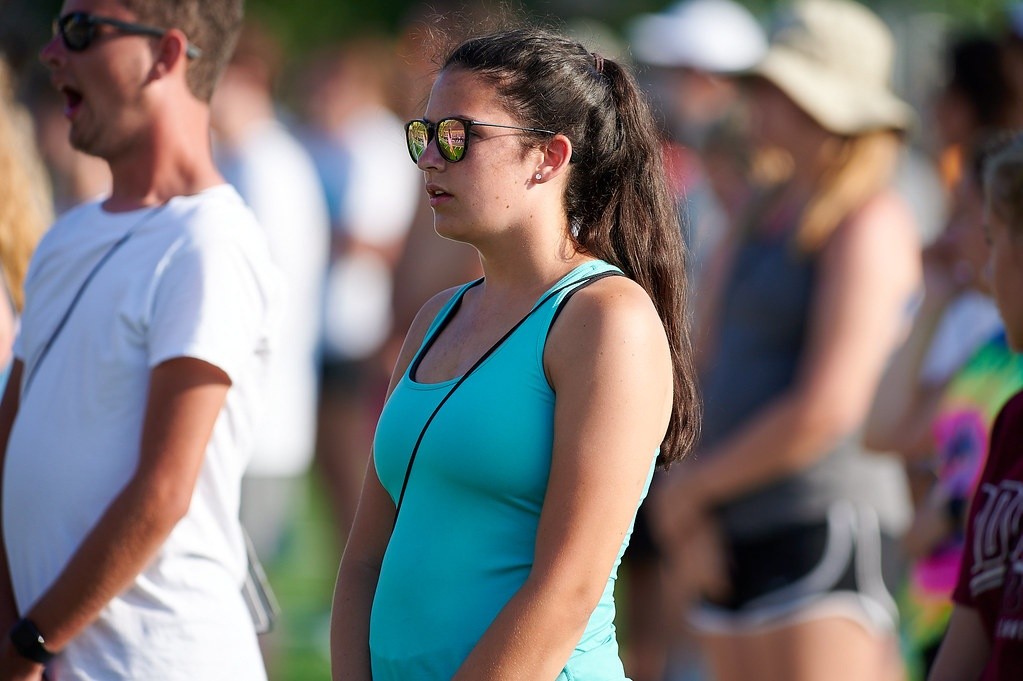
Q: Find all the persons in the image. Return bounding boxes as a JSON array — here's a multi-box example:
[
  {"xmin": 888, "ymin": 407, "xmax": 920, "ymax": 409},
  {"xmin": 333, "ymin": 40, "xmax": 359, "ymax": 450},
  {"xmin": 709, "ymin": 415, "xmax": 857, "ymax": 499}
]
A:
[
  {"xmin": 609, "ymin": 0, "xmax": 1023, "ymax": 681},
  {"xmin": 329, "ymin": 31, "xmax": 701, "ymax": 681},
  {"xmin": 0, "ymin": 0, "xmax": 482, "ymax": 681}
]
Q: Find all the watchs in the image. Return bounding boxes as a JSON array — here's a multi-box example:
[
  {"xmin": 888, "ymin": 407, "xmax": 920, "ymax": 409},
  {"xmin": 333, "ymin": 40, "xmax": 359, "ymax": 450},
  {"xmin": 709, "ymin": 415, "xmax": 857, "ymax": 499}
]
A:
[{"xmin": 10, "ymin": 618, "xmax": 53, "ymax": 663}]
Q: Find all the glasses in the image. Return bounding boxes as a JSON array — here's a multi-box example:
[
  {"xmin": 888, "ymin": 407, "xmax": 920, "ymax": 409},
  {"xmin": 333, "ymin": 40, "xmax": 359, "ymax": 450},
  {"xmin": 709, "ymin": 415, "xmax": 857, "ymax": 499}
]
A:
[
  {"xmin": 403, "ymin": 115, "xmax": 573, "ymax": 166},
  {"xmin": 51, "ymin": 11, "xmax": 202, "ymax": 60}
]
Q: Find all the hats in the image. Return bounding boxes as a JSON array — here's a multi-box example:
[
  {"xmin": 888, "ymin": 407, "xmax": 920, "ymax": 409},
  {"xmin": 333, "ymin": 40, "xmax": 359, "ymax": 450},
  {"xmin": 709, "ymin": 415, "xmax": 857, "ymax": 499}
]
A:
[
  {"xmin": 736, "ymin": 1, "xmax": 918, "ymax": 136},
  {"xmin": 626, "ymin": 0, "xmax": 767, "ymax": 74}
]
